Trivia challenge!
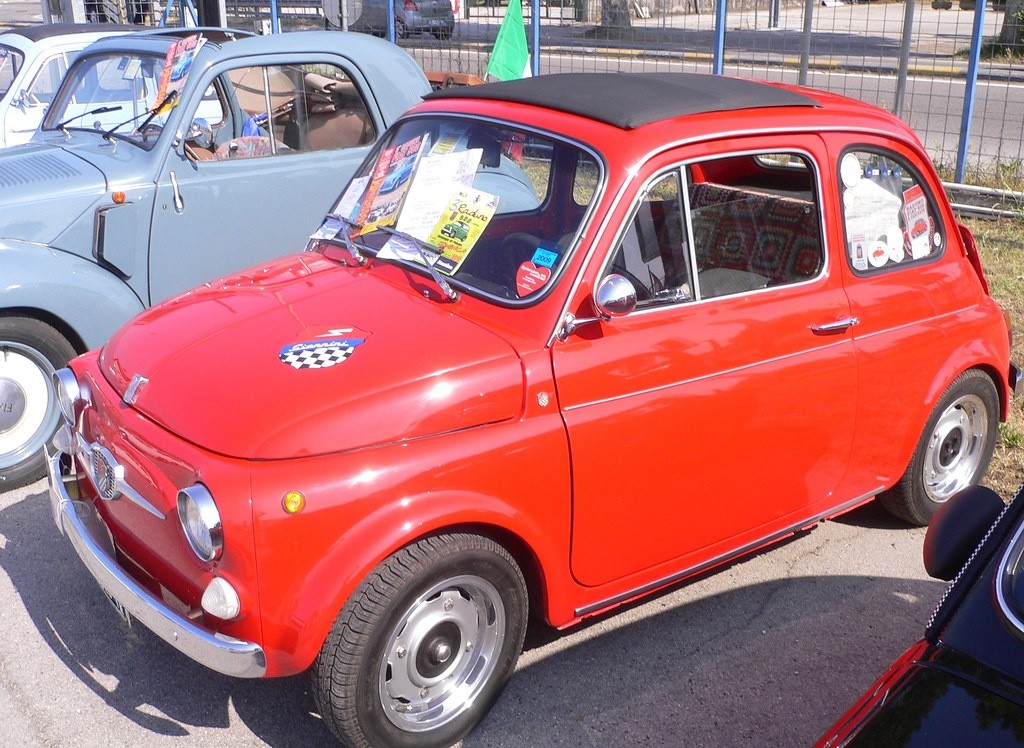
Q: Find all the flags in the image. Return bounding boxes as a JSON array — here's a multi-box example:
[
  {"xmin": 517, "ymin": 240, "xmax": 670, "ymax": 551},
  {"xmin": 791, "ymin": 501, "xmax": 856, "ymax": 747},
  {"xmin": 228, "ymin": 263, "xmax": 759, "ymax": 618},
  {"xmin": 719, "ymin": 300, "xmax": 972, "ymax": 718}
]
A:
[{"xmin": 486, "ymin": 0, "xmax": 532, "ymax": 81}]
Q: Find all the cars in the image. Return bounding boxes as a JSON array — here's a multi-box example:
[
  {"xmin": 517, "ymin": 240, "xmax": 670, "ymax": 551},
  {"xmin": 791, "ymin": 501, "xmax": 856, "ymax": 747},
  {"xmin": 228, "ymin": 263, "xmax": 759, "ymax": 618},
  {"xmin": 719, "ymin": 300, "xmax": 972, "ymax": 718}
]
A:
[
  {"xmin": 0, "ymin": 25, "xmax": 544, "ymax": 493},
  {"xmin": 0, "ymin": 22, "xmax": 225, "ymax": 159},
  {"xmin": 42, "ymin": 71, "xmax": 1024, "ymax": 747},
  {"xmin": 808, "ymin": 486, "xmax": 1024, "ymax": 748},
  {"xmin": 391, "ymin": 0, "xmax": 455, "ymax": 41}
]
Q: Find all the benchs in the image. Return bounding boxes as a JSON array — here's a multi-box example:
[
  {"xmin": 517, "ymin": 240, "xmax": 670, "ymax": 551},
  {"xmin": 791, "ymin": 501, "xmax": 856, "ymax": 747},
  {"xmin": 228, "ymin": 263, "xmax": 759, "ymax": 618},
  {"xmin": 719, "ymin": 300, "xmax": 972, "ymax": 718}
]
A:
[
  {"xmin": 296, "ymin": 83, "xmax": 374, "ymax": 151},
  {"xmin": 645, "ymin": 184, "xmax": 821, "ymax": 285}
]
